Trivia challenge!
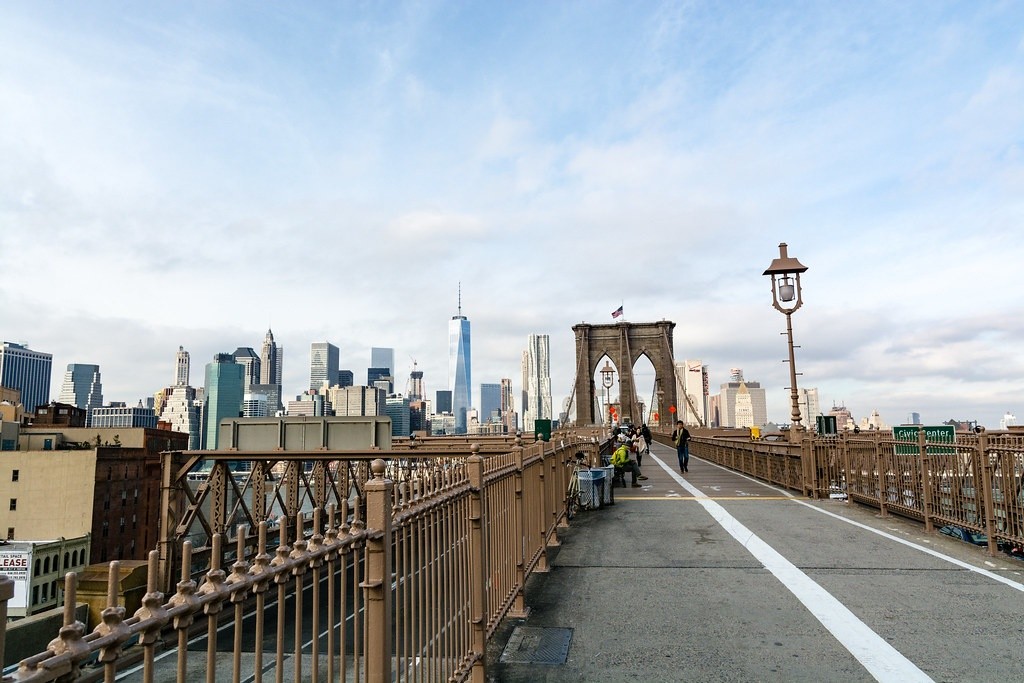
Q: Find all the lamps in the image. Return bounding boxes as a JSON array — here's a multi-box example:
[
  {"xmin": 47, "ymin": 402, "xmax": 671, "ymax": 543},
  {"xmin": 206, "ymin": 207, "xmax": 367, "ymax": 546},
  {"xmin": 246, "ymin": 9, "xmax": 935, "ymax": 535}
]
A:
[
  {"xmin": 409, "ymin": 432, "xmax": 416, "ymax": 441},
  {"xmin": 515, "ymin": 429, "xmax": 522, "ymax": 438}
]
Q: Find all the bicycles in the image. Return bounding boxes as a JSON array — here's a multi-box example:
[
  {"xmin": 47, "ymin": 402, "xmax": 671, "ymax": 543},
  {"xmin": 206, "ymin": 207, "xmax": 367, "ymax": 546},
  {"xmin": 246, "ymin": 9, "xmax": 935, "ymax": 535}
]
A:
[{"xmin": 565, "ymin": 453, "xmax": 594, "ymax": 519}]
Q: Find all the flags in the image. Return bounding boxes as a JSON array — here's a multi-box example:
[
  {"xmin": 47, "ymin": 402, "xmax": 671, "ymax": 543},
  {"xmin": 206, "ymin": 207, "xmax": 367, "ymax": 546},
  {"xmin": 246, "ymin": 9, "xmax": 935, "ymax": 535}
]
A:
[{"xmin": 612, "ymin": 306, "xmax": 623, "ymax": 318}]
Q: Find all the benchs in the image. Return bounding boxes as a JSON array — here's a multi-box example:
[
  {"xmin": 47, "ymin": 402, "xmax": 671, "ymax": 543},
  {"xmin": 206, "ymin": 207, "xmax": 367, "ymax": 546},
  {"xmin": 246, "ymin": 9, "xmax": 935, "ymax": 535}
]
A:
[{"xmin": 603, "ymin": 456, "xmax": 627, "ymax": 488}]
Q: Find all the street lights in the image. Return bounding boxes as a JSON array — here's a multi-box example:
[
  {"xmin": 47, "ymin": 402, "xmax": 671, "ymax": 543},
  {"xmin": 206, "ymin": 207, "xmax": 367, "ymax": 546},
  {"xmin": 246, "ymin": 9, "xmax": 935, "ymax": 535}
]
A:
[
  {"xmin": 599, "ymin": 360, "xmax": 615, "ymax": 439},
  {"xmin": 763, "ymin": 242, "xmax": 808, "ymax": 445}
]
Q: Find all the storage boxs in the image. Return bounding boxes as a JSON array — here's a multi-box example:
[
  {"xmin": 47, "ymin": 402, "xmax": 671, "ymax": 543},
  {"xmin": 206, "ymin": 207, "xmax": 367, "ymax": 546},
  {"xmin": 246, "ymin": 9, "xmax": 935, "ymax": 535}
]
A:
[
  {"xmin": 579, "ymin": 467, "xmax": 614, "ymax": 512},
  {"xmin": 750, "ymin": 426, "xmax": 759, "ymax": 440}
]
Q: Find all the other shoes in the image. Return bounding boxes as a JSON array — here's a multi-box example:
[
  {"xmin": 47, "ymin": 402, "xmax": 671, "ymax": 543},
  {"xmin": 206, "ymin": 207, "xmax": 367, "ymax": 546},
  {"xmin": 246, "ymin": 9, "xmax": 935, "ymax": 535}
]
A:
[
  {"xmin": 681, "ymin": 469, "xmax": 684, "ymax": 474},
  {"xmin": 638, "ymin": 475, "xmax": 648, "ymax": 480},
  {"xmin": 685, "ymin": 466, "xmax": 688, "ymax": 472},
  {"xmin": 631, "ymin": 484, "xmax": 642, "ymax": 488}
]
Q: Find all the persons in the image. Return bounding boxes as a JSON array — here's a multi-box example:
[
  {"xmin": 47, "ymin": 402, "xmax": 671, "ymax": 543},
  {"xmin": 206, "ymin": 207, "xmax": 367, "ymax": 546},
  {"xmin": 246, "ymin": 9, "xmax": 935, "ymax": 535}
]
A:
[
  {"xmin": 671, "ymin": 420, "xmax": 691, "ymax": 473},
  {"xmin": 613, "ymin": 423, "xmax": 652, "ymax": 466},
  {"xmin": 611, "ymin": 440, "xmax": 648, "ymax": 488}
]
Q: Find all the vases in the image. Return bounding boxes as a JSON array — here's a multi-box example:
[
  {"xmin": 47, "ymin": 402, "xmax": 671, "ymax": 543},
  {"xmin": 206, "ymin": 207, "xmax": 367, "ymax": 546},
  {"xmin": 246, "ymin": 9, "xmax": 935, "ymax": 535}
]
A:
[{"xmin": 157, "ymin": 421, "xmax": 172, "ymax": 431}]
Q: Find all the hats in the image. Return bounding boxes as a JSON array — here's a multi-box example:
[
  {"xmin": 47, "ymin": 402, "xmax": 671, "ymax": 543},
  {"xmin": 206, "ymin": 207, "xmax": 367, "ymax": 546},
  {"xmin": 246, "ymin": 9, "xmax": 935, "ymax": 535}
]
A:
[{"xmin": 625, "ymin": 440, "xmax": 633, "ymax": 448}]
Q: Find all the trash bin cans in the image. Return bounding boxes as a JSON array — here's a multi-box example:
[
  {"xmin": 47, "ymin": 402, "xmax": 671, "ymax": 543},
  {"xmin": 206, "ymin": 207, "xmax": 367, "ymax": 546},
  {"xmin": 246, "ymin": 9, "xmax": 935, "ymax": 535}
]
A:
[
  {"xmin": 578, "ymin": 469, "xmax": 607, "ymax": 510},
  {"xmin": 598, "ymin": 466, "xmax": 615, "ymax": 505}
]
